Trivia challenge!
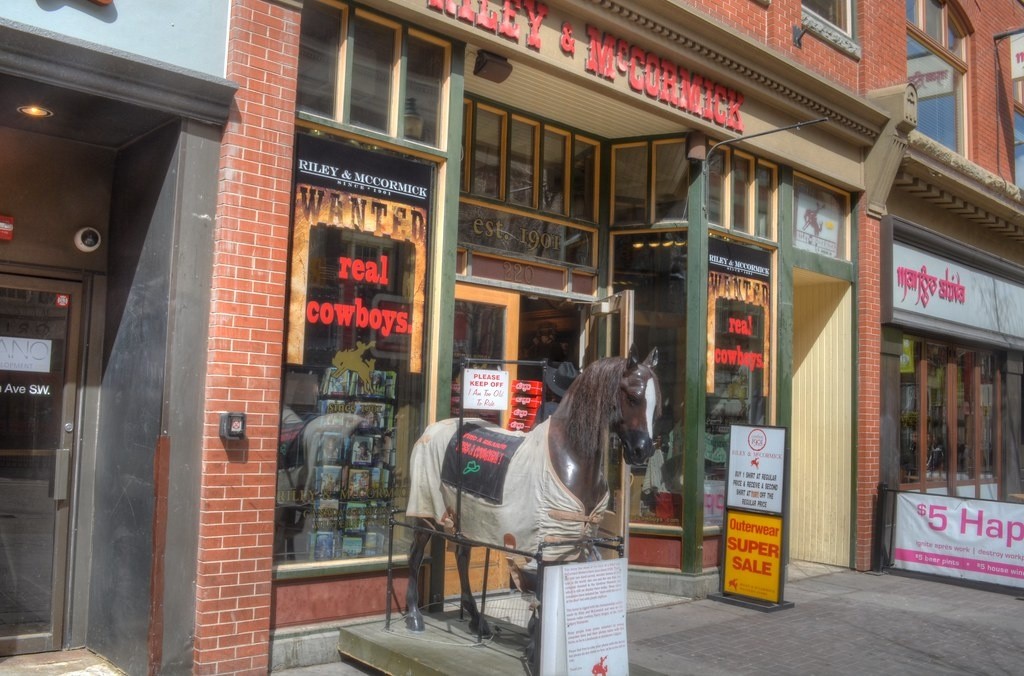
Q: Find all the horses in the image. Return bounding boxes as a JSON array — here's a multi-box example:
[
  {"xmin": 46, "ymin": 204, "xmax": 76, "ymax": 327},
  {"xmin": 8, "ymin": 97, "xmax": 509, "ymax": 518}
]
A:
[{"xmin": 403, "ymin": 340, "xmax": 666, "ymax": 644}]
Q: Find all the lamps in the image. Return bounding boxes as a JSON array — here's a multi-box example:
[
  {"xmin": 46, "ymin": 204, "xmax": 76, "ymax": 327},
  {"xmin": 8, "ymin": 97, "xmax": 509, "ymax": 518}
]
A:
[
  {"xmin": 405, "ymin": 98, "xmax": 423, "ymax": 139},
  {"xmin": 674, "ymin": 233, "xmax": 685, "ymax": 245},
  {"xmin": 473, "ymin": 47, "xmax": 513, "ymax": 84},
  {"xmin": 632, "ymin": 235, "xmax": 644, "ymax": 249},
  {"xmin": 661, "ymin": 232, "xmax": 673, "ymax": 246},
  {"xmin": 648, "ymin": 233, "xmax": 660, "ymax": 247}
]
